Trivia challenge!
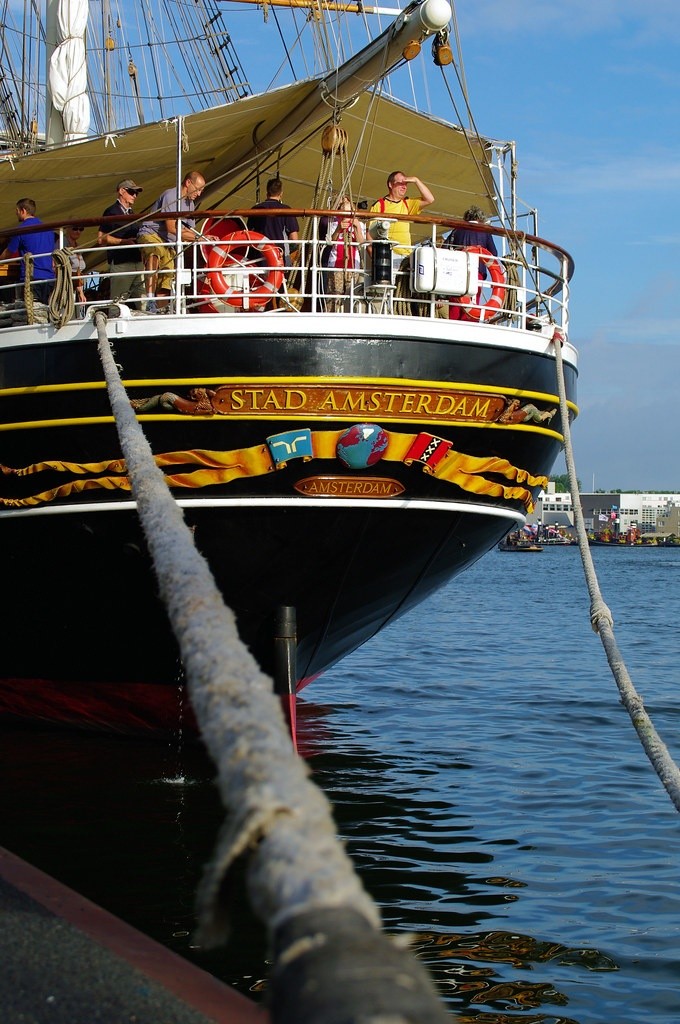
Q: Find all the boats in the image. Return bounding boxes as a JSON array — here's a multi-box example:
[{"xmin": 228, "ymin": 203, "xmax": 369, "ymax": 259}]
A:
[
  {"xmin": 587, "ymin": 517, "xmax": 680, "ymax": 547},
  {"xmin": 0, "ymin": 1, "xmax": 581, "ymax": 826},
  {"xmin": 540, "ymin": 533, "xmax": 570, "ymax": 545},
  {"xmin": 499, "ymin": 527, "xmax": 544, "ymax": 552}
]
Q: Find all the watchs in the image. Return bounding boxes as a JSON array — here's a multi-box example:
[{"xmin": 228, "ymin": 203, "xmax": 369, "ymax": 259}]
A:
[{"xmin": 195, "ymin": 234, "xmax": 199, "ymax": 241}]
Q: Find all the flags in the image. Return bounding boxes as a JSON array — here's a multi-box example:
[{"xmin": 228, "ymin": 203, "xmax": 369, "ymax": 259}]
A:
[{"xmin": 598, "ymin": 513, "xmax": 609, "ymax": 522}]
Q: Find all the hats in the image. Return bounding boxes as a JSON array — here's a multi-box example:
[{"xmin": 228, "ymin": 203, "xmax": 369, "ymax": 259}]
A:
[{"xmin": 117, "ymin": 179, "xmax": 144, "ymax": 193}]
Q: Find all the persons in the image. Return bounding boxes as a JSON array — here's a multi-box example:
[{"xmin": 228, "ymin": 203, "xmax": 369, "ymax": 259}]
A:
[
  {"xmin": 135, "ymin": 171, "xmax": 220, "ymax": 315},
  {"xmin": 429, "ymin": 205, "xmax": 498, "ymax": 280},
  {"xmin": 246, "ymin": 178, "xmax": 300, "ymax": 256},
  {"xmin": 364, "ymin": 171, "xmax": 435, "ymax": 256},
  {"xmin": 55, "ymin": 214, "xmax": 87, "ymax": 306},
  {"xmin": 0, "ymin": 197, "xmax": 55, "ymax": 305},
  {"xmin": 324, "ymin": 196, "xmax": 366, "ymax": 313},
  {"xmin": 98, "ymin": 178, "xmax": 149, "ymax": 318}
]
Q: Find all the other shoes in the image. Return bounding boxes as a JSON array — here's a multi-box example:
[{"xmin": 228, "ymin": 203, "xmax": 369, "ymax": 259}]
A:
[{"xmin": 146, "ymin": 301, "xmax": 159, "ymax": 313}]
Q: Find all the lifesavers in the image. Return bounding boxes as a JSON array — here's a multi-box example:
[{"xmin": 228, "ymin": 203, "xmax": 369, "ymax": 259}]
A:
[
  {"xmin": 207, "ymin": 229, "xmax": 285, "ymax": 307},
  {"xmin": 455, "ymin": 244, "xmax": 507, "ymax": 319}
]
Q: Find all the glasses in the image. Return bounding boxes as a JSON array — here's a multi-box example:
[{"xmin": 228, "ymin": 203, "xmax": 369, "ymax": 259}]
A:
[
  {"xmin": 124, "ymin": 188, "xmax": 138, "ymax": 197},
  {"xmin": 71, "ymin": 227, "xmax": 84, "ymax": 231}
]
